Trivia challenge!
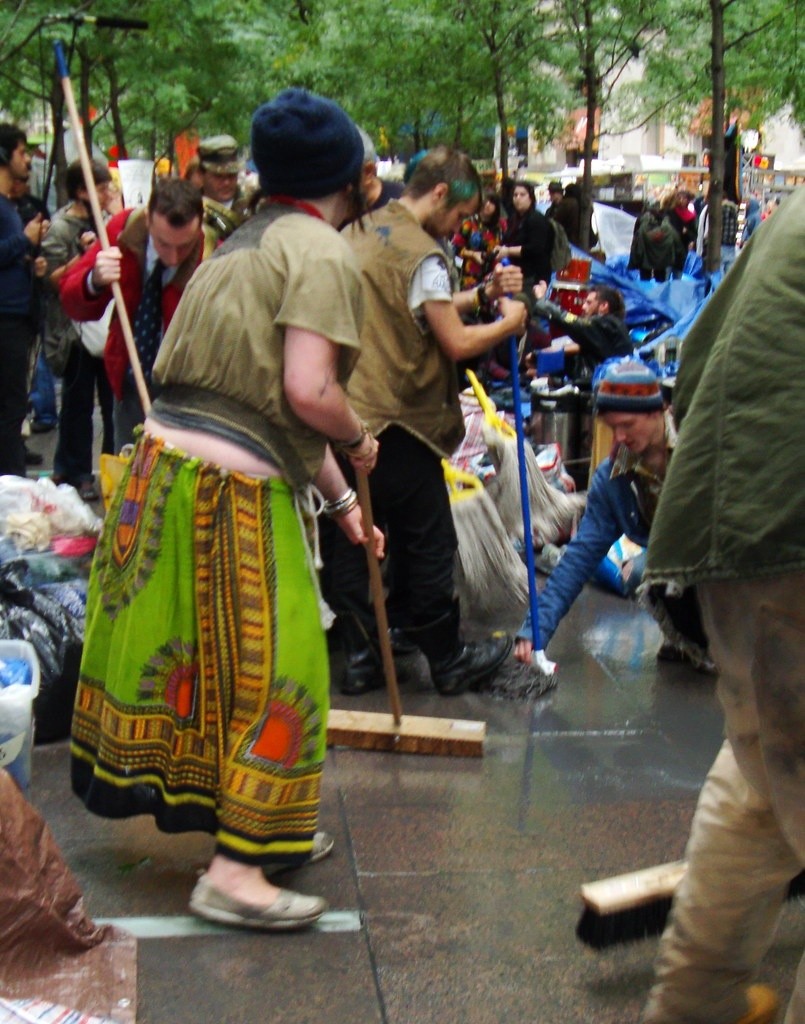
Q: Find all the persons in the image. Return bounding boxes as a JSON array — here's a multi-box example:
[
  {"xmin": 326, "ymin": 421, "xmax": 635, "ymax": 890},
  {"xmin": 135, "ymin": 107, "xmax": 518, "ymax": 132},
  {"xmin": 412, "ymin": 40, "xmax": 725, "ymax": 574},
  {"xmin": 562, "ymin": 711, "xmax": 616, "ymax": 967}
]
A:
[
  {"xmin": 1, "ymin": 119, "xmax": 774, "ymax": 501},
  {"xmin": 319, "ymin": 144, "xmax": 511, "ymax": 696},
  {"xmin": 511, "ymin": 356, "xmax": 719, "ymax": 676},
  {"xmin": 65, "ymin": 93, "xmax": 384, "ymax": 929},
  {"xmin": 640, "ymin": 184, "xmax": 805, "ymax": 1024}
]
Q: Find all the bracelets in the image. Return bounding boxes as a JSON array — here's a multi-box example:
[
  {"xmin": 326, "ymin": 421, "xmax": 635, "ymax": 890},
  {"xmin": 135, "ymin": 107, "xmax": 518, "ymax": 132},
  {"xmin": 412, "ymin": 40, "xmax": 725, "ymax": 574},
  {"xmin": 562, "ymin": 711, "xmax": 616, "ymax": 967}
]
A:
[
  {"xmin": 336, "ymin": 416, "xmax": 366, "ymax": 449},
  {"xmin": 478, "ymin": 280, "xmax": 492, "ymax": 315},
  {"xmin": 322, "ymin": 488, "xmax": 358, "ymax": 522},
  {"xmin": 343, "ymin": 427, "xmax": 375, "ymax": 469}
]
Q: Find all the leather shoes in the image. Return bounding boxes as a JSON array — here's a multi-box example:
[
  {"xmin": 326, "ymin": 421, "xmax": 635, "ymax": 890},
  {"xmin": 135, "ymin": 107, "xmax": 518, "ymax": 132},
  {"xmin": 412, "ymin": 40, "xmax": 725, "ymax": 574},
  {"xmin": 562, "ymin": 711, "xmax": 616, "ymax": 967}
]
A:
[
  {"xmin": 383, "ymin": 626, "xmax": 416, "ymax": 653},
  {"xmin": 341, "ymin": 641, "xmax": 412, "ymax": 696},
  {"xmin": 431, "ymin": 630, "xmax": 512, "ymax": 696}
]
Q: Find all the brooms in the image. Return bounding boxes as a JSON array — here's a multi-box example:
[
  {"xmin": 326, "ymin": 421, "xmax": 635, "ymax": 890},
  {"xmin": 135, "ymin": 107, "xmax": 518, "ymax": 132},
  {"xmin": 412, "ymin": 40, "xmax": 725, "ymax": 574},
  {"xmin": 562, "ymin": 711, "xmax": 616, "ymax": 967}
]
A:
[
  {"xmin": 573, "ymin": 857, "xmax": 805, "ymax": 952},
  {"xmin": 327, "ymin": 464, "xmax": 486, "ymax": 759}
]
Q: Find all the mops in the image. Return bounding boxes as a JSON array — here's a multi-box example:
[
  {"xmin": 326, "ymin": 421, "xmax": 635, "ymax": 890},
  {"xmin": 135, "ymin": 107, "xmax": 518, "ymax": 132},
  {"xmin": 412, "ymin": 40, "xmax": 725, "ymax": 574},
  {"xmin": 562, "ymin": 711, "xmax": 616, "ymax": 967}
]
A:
[
  {"xmin": 490, "ymin": 256, "xmax": 560, "ymax": 704},
  {"xmin": 442, "ymin": 458, "xmax": 531, "ymax": 619},
  {"xmin": 464, "ymin": 368, "xmax": 587, "ymax": 543}
]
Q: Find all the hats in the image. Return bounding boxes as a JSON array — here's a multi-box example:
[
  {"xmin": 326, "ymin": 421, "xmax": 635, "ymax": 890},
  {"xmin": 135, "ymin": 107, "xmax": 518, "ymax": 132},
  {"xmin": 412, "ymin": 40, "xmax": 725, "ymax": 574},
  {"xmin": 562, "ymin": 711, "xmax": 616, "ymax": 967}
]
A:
[
  {"xmin": 595, "ymin": 359, "xmax": 665, "ymax": 412},
  {"xmin": 197, "ymin": 134, "xmax": 244, "ymax": 174}
]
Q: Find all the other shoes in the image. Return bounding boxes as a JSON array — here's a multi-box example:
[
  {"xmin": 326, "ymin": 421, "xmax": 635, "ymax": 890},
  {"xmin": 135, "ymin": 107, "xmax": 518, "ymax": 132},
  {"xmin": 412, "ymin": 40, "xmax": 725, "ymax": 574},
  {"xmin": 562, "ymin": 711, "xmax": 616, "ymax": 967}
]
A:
[
  {"xmin": 187, "ymin": 875, "xmax": 328, "ymax": 930},
  {"xmin": 262, "ymin": 832, "xmax": 337, "ymax": 874},
  {"xmin": 79, "ymin": 481, "xmax": 98, "ymax": 501},
  {"xmin": 20, "ymin": 442, "xmax": 43, "ymax": 466},
  {"xmin": 30, "ymin": 421, "xmax": 58, "ymax": 433},
  {"xmin": 742, "ymin": 981, "xmax": 780, "ymax": 1022}
]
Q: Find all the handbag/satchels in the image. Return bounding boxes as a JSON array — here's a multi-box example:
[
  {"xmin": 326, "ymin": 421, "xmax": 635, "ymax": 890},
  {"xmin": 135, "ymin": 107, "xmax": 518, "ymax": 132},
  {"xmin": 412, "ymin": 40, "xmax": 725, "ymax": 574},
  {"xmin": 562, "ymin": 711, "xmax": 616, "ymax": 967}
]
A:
[{"xmin": 42, "ymin": 300, "xmax": 86, "ymax": 383}]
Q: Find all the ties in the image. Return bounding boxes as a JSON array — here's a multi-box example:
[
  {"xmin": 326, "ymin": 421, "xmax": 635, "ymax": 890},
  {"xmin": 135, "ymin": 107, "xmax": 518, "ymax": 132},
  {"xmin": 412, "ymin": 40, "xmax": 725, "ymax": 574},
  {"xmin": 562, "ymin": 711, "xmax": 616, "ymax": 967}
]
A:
[{"xmin": 128, "ymin": 257, "xmax": 170, "ymax": 397}]
